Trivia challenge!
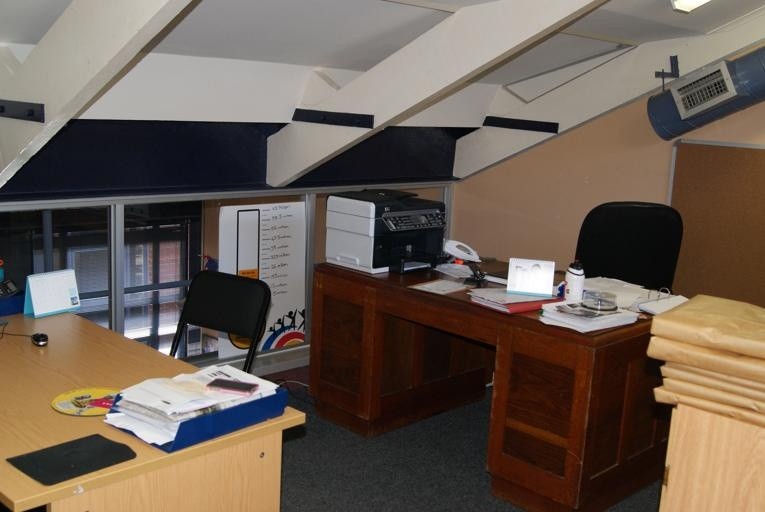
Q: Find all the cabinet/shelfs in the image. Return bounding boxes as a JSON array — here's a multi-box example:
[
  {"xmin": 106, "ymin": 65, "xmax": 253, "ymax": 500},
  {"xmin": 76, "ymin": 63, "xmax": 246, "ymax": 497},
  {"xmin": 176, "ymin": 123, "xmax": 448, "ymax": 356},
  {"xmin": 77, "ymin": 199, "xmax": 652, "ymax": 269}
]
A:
[{"xmin": 658, "ymin": 403, "xmax": 765, "ymax": 511}]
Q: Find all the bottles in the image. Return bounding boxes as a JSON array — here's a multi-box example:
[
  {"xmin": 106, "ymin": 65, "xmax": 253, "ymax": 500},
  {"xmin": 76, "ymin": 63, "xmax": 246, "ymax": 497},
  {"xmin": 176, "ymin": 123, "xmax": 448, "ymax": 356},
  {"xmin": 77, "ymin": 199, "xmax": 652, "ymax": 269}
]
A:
[{"xmin": 564, "ymin": 258, "xmax": 584, "ymax": 302}]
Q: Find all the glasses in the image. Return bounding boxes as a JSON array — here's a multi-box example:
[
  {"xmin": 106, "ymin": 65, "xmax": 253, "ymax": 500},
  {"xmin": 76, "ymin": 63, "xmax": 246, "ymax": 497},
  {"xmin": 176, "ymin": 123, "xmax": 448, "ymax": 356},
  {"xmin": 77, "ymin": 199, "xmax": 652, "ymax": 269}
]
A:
[{"xmin": 646, "ymin": 288, "xmax": 669, "ymax": 302}]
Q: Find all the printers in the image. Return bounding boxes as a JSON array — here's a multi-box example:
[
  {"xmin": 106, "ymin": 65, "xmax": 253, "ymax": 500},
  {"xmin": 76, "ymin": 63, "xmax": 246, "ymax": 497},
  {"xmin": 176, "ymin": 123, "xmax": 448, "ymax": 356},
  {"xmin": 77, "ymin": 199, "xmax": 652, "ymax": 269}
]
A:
[{"xmin": 325, "ymin": 188, "xmax": 446, "ymax": 275}]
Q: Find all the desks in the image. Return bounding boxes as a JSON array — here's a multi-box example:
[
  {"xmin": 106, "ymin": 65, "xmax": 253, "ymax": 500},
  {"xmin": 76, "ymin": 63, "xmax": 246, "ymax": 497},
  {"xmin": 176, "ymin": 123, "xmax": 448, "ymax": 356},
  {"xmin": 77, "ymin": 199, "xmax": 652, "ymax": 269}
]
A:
[
  {"xmin": 0, "ymin": 310, "xmax": 305, "ymax": 510},
  {"xmin": 310, "ymin": 254, "xmax": 688, "ymax": 511}
]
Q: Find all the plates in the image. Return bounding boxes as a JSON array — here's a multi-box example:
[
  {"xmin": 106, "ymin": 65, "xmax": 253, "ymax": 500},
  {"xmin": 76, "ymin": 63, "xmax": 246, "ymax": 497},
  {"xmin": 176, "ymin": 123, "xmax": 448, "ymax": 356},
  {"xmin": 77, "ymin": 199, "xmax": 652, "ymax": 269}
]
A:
[{"xmin": 51, "ymin": 386, "xmax": 123, "ymax": 416}]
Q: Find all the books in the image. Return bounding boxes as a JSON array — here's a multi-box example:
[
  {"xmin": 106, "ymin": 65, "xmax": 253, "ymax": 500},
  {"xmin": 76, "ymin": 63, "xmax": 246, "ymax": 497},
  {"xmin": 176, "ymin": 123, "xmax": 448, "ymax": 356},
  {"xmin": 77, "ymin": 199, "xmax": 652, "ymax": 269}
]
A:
[{"xmin": 466, "ymin": 278, "xmax": 690, "ymax": 334}]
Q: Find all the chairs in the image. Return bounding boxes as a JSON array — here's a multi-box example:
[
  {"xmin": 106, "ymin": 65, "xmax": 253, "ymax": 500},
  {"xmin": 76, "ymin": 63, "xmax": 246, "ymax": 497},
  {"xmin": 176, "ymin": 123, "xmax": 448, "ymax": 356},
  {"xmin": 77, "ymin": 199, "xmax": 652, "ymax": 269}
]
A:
[
  {"xmin": 572, "ymin": 200, "xmax": 683, "ymax": 293},
  {"xmin": 166, "ymin": 269, "xmax": 271, "ymax": 374}
]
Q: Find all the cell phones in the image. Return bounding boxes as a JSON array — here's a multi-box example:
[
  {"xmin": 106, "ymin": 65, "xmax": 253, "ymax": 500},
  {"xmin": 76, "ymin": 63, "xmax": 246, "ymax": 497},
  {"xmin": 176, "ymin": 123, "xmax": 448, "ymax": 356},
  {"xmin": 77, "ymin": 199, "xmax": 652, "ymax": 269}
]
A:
[{"xmin": 206, "ymin": 379, "xmax": 258, "ymax": 396}]
